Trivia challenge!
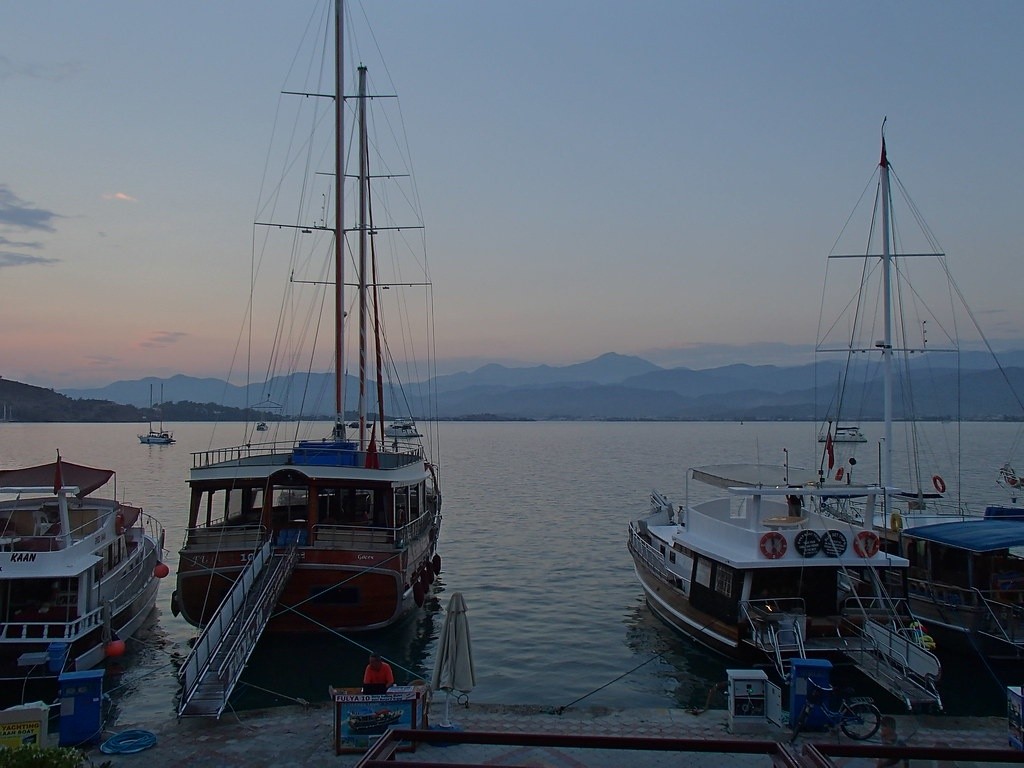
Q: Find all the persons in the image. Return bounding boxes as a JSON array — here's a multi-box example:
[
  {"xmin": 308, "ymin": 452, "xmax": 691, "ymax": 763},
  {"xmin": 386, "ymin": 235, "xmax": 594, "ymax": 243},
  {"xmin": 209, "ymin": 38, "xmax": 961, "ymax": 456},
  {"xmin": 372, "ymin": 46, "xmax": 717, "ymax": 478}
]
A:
[{"xmin": 364, "ymin": 652, "xmax": 394, "ymax": 687}]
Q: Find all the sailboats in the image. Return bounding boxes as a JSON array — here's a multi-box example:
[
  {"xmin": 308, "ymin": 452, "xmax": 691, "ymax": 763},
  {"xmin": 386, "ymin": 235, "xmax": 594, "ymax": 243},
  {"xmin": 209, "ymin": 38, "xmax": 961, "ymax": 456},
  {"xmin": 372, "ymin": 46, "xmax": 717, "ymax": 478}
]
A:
[
  {"xmin": 169, "ymin": 0, "xmax": 445, "ymax": 719},
  {"xmin": 809, "ymin": 117, "xmax": 1024, "ymax": 668}
]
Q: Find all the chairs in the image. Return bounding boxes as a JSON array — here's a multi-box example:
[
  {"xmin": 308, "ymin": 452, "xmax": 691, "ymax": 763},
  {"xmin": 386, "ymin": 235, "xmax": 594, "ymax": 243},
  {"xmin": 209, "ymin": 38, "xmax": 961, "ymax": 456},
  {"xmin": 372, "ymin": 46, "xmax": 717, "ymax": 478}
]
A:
[
  {"xmin": 0, "ymin": 518, "xmax": 16, "ymax": 536},
  {"xmin": 17, "ymin": 523, "xmax": 60, "ymax": 552}
]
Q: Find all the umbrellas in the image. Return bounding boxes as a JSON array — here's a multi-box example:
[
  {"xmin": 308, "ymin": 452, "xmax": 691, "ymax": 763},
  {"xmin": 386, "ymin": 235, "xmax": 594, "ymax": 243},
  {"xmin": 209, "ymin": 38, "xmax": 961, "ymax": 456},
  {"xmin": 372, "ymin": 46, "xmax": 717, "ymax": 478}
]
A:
[{"xmin": 430, "ymin": 592, "xmax": 476, "ymax": 728}]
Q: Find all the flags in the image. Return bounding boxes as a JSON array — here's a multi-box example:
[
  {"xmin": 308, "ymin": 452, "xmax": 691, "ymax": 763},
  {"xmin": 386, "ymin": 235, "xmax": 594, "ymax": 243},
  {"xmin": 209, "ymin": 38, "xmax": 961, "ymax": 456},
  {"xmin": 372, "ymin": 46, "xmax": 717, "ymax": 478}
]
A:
[
  {"xmin": 53, "ymin": 455, "xmax": 63, "ymax": 496},
  {"xmin": 826, "ymin": 432, "xmax": 834, "ymax": 469},
  {"xmin": 366, "ymin": 422, "xmax": 379, "ymax": 469}
]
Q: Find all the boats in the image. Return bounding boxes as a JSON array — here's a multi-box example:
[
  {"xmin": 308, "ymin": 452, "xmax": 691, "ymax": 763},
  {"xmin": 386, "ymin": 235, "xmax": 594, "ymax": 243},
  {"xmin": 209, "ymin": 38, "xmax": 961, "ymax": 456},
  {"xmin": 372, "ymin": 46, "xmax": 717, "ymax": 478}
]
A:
[
  {"xmin": 137, "ymin": 430, "xmax": 176, "ymax": 444},
  {"xmin": 257, "ymin": 422, "xmax": 268, "ymax": 431},
  {"xmin": 384, "ymin": 419, "xmax": 423, "ymax": 437},
  {"xmin": 338, "ymin": 421, "xmax": 348, "ymax": 426},
  {"xmin": 818, "ymin": 426, "xmax": 868, "ymax": 442},
  {"xmin": 0, "ymin": 448, "xmax": 169, "ymax": 727},
  {"xmin": 349, "ymin": 421, "xmax": 373, "ymax": 428},
  {"xmin": 627, "ymin": 462, "xmax": 947, "ymax": 712}
]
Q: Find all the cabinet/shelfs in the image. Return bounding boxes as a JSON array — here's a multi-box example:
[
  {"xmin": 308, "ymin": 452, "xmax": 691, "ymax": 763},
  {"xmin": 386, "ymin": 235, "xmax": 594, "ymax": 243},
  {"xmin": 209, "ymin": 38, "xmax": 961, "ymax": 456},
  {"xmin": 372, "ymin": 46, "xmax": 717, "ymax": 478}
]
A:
[{"xmin": 726, "ymin": 669, "xmax": 768, "ymax": 731}]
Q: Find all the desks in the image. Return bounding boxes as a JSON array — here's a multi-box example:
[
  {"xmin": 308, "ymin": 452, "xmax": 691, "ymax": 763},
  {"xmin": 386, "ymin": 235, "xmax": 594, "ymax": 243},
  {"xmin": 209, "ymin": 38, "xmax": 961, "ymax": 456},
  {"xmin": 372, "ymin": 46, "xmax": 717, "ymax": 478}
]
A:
[
  {"xmin": 762, "ymin": 515, "xmax": 808, "ymax": 531},
  {"xmin": 0, "ymin": 537, "xmax": 22, "ymax": 552}
]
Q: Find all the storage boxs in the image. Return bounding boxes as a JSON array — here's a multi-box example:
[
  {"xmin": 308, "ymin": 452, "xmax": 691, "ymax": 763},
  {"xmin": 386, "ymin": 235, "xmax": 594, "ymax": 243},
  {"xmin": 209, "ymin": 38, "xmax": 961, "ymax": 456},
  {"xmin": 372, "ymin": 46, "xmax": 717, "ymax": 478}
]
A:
[
  {"xmin": 293, "ymin": 442, "xmax": 358, "ymax": 466},
  {"xmin": 278, "ymin": 529, "xmax": 308, "ymax": 545}
]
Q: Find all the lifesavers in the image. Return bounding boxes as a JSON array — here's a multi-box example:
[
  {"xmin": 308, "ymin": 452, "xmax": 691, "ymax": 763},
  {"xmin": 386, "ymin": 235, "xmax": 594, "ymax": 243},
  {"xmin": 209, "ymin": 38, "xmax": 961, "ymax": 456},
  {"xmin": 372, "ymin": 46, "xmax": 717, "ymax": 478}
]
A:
[
  {"xmin": 412, "ymin": 554, "xmax": 441, "ymax": 608},
  {"xmin": 853, "ymin": 530, "xmax": 881, "ymax": 559},
  {"xmin": 760, "ymin": 531, "xmax": 789, "ymax": 559},
  {"xmin": 933, "ymin": 474, "xmax": 946, "ymax": 493},
  {"xmin": 889, "ymin": 510, "xmax": 904, "ymax": 535},
  {"xmin": 793, "ymin": 529, "xmax": 848, "ymax": 558},
  {"xmin": 835, "ymin": 467, "xmax": 845, "ymax": 481}
]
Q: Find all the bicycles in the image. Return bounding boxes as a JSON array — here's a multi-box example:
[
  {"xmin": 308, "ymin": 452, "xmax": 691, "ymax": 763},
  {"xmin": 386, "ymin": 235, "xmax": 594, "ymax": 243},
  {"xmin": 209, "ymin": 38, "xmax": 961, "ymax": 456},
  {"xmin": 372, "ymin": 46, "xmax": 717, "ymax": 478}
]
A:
[{"xmin": 788, "ymin": 666, "xmax": 884, "ymax": 744}]
